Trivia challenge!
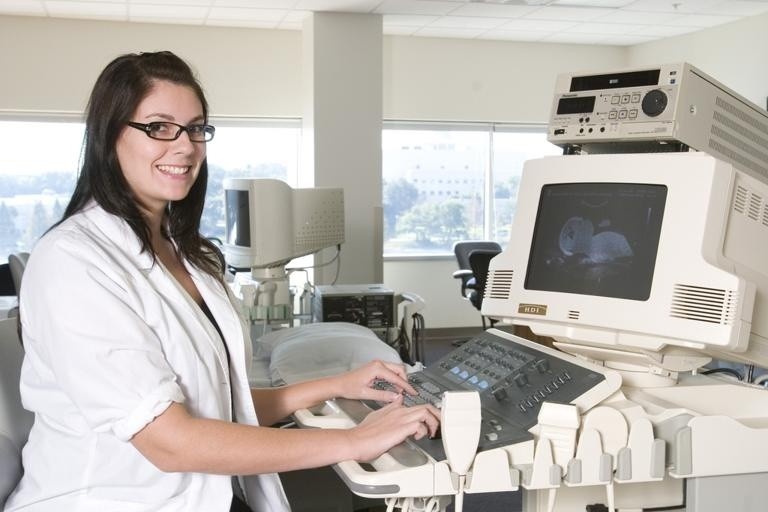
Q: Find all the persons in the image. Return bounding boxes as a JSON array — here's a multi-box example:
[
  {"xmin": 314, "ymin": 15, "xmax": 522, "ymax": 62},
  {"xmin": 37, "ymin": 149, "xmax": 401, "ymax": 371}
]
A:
[
  {"xmin": 559, "ymin": 216, "xmax": 634, "ymax": 263},
  {"xmin": 0, "ymin": 51, "xmax": 440, "ymax": 512}
]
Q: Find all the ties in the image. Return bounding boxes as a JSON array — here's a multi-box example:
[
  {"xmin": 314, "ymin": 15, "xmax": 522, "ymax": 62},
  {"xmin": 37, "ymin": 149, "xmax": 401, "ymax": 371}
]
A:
[
  {"xmin": 481, "ymin": 151, "xmax": 764, "ymax": 388},
  {"xmin": 222, "ymin": 177, "xmax": 345, "ymax": 277}
]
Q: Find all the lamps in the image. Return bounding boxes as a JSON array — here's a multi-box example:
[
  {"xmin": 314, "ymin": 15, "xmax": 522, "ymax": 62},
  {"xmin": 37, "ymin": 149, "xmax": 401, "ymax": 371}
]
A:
[
  {"xmin": 451, "ymin": 241, "xmax": 502, "ymax": 345},
  {"xmin": 464, "ymin": 249, "xmax": 502, "ymax": 330}
]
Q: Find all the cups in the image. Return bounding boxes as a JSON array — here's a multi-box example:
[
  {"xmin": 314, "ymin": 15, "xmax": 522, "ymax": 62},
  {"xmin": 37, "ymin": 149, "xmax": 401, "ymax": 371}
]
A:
[{"xmin": 125, "ymin": 121, "xmax": 215, "ymax": 143}]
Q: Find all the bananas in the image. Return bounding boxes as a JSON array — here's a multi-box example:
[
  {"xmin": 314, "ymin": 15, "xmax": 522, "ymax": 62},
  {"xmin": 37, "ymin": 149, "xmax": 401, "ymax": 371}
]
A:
[{"xmin": 356, "ymin": 370, "xmax": 531, "ymax": 466}]
